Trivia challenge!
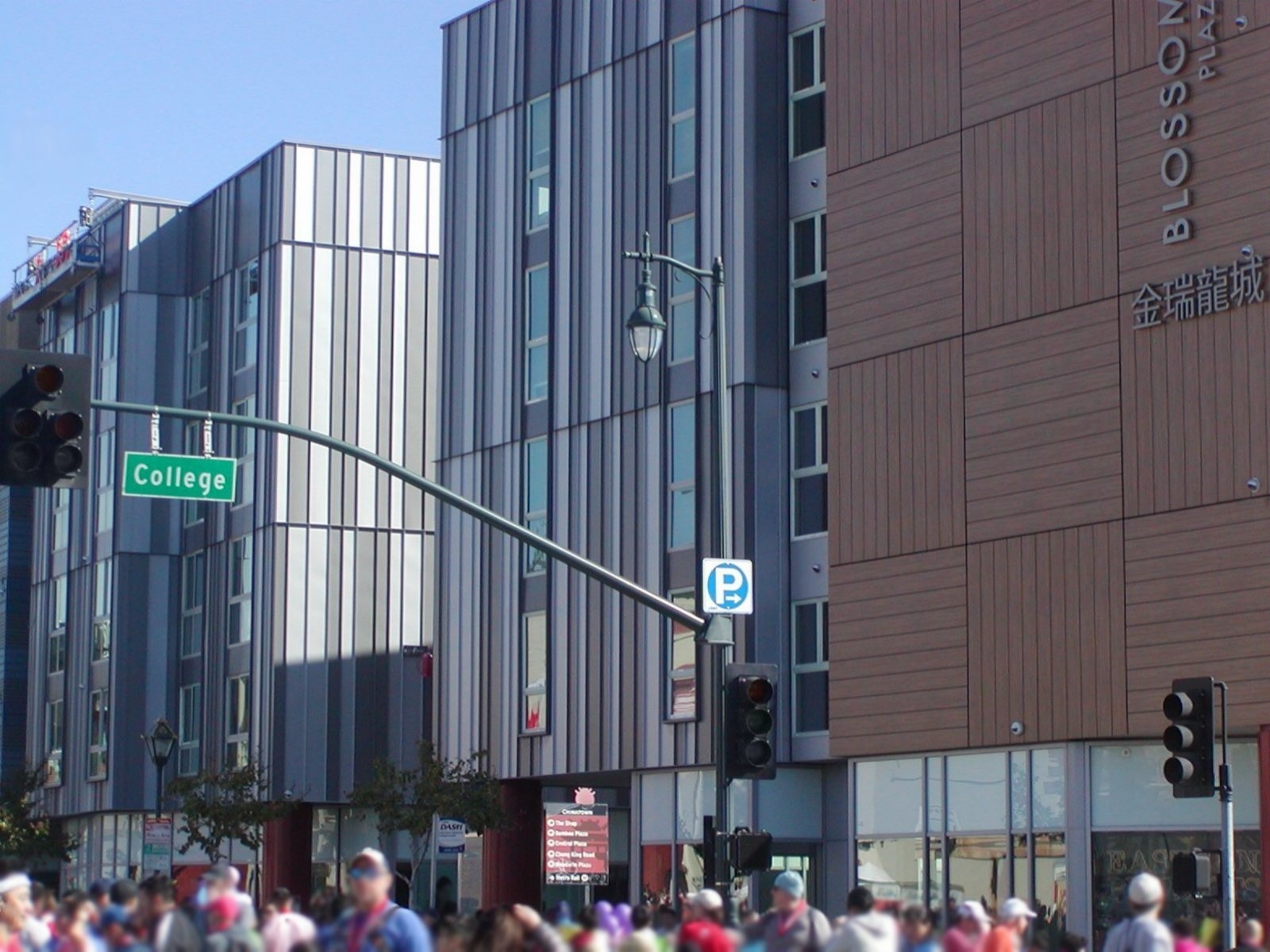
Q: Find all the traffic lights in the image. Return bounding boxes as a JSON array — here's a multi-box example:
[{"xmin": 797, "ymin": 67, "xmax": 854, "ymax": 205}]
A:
[
  {"xmin": 1172, "ymin": 853, "xmax": 1210, "ymax": 893},
  {"xmin": 1162, "ymin": 675, "xmax": 1215, "ymax": 798},
  {"xmin": 725, "ymin": 663, "xmax": 779, "ymax": 781},
  {"xmin": 0, "ymin": 349, "xmax": 90, "ymax": 489},
  {"xmin": 730, "ymin": 833, "xmax": 773, "ymax": 873}
]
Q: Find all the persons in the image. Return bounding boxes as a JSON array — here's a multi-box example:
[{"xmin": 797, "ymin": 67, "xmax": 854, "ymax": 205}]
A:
[{"xmin": 0, "ymin": 844, "xmax": 1264, "ymax": 952}]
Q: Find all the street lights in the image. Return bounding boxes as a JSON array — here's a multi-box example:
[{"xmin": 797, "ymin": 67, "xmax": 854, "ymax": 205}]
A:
[
  {"xmin": 621, "ymin": 230, "xmax": 739, "ymax": 927},
  {"xmin": 143, "ymin": 717, "xmax": 178, "ymax": 874}
]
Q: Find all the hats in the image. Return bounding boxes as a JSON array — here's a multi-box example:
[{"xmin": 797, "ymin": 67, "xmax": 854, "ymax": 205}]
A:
[
  {"xmin": 201, "ymin": 863, "xmax": 230, "ymax": 880},
  {"xmin": 691, "ymin": 888, "xmax": 724, "ymax": 913},
  {"xmin": 996, "ymin": 898, "xmax": 1038, "ymax": 918},
  {"xmin": 957, "ymin": 900, "xmax": 995, "ymax": 922},
  {"xmin": 774, "ymin": 871, "xmax": 805, "ymax": 899},
  {"xmin": 1239, "ymin": 918, "xmax": 1264, "ymax": 938},
  {"xmin": 1128, "ymin": 872, "xmax": 1164, "ymax": 905},
  {"xmin": 349, "ymin": 846, "xmax": 390, "ymax": 876},
  {"xmin": 93, "ymin": 904, "xmax": 129, "ymax": 931}
]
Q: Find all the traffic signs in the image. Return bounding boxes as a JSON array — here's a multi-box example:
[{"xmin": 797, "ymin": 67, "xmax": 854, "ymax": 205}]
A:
[{"xmin": 122, "ymin": 451, "xmax": 236, "ymax": 503}]
